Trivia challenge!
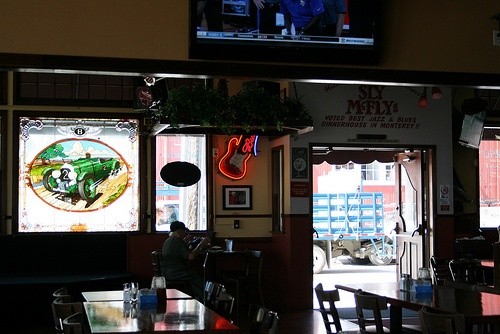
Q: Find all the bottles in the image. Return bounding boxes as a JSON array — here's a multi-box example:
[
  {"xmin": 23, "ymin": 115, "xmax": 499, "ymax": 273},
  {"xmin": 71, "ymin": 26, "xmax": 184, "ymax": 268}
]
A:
[
  {"xmin": 400, "ymin": 274, "xmax": 410, "ymax": 292},
  {"xmin": 123, "ymin": 282, "xmax": 138, "ymax": 303}
]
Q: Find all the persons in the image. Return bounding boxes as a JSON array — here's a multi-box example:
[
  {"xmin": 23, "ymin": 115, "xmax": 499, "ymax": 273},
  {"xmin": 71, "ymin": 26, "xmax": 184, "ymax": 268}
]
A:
[
  {"xmin": 162, "ymin": 221, "xmax": 209, "ymax": 286},
  {"xmin": 197, "ymin": 0, "xmax": 265, "ymax": 32}
]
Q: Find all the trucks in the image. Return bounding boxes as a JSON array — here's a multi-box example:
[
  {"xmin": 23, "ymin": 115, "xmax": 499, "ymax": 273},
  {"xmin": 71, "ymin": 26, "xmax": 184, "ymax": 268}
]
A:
[{"xmin": 313, "ymin": 192, "xmax": 384, "ymax": 275}]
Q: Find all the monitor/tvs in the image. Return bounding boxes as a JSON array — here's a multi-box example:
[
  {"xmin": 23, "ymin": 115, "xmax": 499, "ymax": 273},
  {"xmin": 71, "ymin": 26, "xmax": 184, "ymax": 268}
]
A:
[
  {"xmin": 188, "ymin": 0, "xmax": 389, "ymax": 66},
  {"xmin": 458, "ymin": 114, "xmax": 484, "ymax": 149}
]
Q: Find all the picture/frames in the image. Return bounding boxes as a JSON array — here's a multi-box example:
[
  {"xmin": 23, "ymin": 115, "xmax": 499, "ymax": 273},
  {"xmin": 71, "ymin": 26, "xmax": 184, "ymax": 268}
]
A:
[{"xmin": 222, "ymin": 185, "xmax": 253, "ymax": 210}]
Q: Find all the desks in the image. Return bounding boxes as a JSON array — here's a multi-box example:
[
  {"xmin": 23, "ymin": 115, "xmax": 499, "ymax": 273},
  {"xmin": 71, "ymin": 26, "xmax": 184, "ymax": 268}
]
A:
[
  {"xmin": 335, "ymin": 279, "xmax": 500, "ymax": 334},
  {"xmin": 81, "ymin": 289, "xmax": 193, "ymax": 302},
  {"xmin": 83, "ymin": 298, "xmax": 239, "ymax": 334}
]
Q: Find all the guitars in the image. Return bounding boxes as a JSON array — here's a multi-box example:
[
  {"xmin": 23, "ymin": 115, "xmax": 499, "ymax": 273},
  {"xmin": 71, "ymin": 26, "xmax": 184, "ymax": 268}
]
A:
[{"xmin": 217, "ymin": 134, "xmax": 256, "ymax": 181}]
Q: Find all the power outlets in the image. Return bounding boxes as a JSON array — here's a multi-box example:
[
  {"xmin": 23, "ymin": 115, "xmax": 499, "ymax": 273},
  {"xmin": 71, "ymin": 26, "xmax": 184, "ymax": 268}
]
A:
[{"xmin": 493, "ymin": 30, "xmax": 500, "ymax": 46}]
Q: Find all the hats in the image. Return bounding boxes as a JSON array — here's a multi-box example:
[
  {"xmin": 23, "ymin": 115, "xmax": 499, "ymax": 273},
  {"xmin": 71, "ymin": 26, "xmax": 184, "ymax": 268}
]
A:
[{"xmin": 170, "ymin": 221, "xmax": 186, "ymax": 233}]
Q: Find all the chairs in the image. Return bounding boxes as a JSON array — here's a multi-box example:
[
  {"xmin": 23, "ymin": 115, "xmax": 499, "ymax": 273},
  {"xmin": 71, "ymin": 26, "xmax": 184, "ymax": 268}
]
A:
[
  {"xmin": 449, "ymin": 261, "xmax": 459, "ymax": 282},
  {"xmin": 52, "ymin": 288, "xmax": 83, "ymax": 334},
  {"xmin": 315, "ymin": 283, "xmax": 390, "ymax": 334},
  {"xmin": 254, "ymin": 308, "xmax": 279, "ymax": 334},
  {"xmin": 431, "ymin": 255, "xmax": 441, "ymax": 281},
  {"xmin": 201, "ymin": 281, "xmax": 238, "ymax": 320},
  {"xmin": 354, "ymin": 289, "xmax": 388, "ymax": 334}
]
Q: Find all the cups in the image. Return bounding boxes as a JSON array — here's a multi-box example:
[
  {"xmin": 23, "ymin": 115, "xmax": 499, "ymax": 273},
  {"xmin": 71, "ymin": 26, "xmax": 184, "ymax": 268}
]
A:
[
  {"xmin": 226, "ymin": 240, "xmax": 233, "ymax": 252},
  {"xmin": 152, "ymin": 276, "xmax": 167, "ymax": 312}
]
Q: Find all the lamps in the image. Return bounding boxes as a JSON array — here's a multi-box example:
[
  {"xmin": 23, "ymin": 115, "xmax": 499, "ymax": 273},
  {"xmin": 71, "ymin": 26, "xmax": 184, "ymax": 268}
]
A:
[
  {"xmin": 418, "ymin": 87, "xmax": 428, "ymax": 109},
  {"xmin": 432, "ymin": 87, "xmax": 442, "ymax": 100}
]
